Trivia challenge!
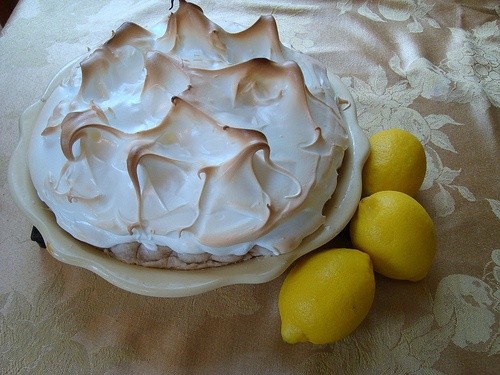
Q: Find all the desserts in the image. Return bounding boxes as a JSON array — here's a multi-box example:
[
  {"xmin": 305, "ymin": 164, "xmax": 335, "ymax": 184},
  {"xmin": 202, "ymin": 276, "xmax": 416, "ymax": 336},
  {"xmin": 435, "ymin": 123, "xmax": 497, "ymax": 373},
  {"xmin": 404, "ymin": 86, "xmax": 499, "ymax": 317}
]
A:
[{"xmin": 26, "ymin": 1, "xmax": 351, "ymax": 272}]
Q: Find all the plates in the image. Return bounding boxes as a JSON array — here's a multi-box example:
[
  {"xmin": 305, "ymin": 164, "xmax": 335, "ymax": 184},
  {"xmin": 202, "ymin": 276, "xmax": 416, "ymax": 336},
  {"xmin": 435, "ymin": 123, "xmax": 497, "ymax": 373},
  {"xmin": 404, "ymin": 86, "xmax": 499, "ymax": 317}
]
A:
[{"xmin": 6, "ymin": 14, "xmax": 372, "ymax": 298}]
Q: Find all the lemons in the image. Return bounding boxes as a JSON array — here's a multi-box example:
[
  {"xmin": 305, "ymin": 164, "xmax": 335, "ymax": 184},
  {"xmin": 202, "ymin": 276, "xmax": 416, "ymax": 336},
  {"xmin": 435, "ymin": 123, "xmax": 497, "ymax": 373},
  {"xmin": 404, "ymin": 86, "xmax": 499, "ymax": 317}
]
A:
[
  {"xmin": 348, "ymin": 189, "xmax": 437, "ymax": 282},
  {"xmin": 360, "ymin": 128, "xmax": 428, "ymax": 198},
  {"xmin": 277, "ymin": 247, "xmax": 377, "ymax": 346}
]
